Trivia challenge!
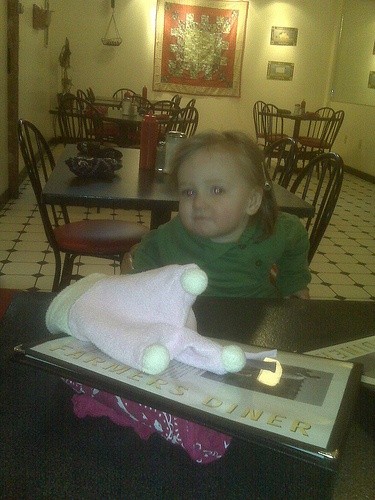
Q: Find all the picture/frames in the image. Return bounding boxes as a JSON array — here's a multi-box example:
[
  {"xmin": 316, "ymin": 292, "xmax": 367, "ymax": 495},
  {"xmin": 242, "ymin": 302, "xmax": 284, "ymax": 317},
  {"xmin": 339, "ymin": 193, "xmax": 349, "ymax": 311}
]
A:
[
  {"xmin": 269, "ymin": 27, "xmax": 298, "ymax": 46},
  {"xmin": 266, "ymin": 61, "xmax": 294, "ymax": 82}
]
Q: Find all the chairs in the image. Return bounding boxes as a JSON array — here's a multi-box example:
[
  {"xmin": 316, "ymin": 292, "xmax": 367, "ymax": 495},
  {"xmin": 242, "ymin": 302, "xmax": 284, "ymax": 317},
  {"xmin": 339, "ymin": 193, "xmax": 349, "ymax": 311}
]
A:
[{"xmin": 16, "ymin": 88, "xmax": 345, "ymax": 292}]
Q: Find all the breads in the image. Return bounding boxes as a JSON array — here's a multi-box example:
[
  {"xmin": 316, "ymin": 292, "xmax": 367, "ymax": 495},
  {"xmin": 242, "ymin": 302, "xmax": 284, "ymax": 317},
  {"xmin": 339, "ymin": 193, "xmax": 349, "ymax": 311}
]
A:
[{"xmin": 65, "ymin": 141, "xmax": 122, "ymax": 182}]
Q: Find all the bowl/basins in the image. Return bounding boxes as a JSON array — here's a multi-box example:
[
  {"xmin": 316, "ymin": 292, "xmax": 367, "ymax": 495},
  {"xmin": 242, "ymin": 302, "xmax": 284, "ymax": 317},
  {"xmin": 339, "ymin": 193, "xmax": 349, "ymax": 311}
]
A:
[
  {"xmin": 64, "ymin": 157, "xmax": 123, "ymax": 181},
  {"xmin": 277, "ymin": 109, "xmax": 291, "ymax": 114}
]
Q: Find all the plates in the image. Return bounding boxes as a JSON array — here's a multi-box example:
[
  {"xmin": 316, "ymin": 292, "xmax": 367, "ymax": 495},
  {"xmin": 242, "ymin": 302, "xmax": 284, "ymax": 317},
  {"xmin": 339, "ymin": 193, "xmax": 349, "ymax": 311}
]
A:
[{"xmin": 76, "ymin": 141, "xmax": 123, "ymax": 159}]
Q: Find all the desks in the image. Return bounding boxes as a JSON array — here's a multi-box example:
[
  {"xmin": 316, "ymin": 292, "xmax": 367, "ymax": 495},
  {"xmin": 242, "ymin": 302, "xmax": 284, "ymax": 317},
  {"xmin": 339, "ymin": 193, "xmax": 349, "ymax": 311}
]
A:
[
  {"xmin": 0, "ymin": 291, "xmax": 375, "ymax": 500},
  {"xmin": 81, "ymin": 110, "xmax": 195, "ymax": 148},
  {"xmin": 258, "ymin": 111, "xmax": 341, "ymax": 139},
  {"xmin": 40, "ymin": 145, "xmax": 315, "ymax": 231},
  {"xmin": 92, "ymin": 100, "xmax": 122, "ymax": 107}
]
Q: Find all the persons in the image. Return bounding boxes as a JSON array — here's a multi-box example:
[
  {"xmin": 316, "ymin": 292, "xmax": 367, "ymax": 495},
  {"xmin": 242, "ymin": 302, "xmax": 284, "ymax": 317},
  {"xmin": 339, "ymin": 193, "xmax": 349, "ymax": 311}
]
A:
[{"xmin": 133, "ymin": 131, "xmax": 311, "ymax": 299}]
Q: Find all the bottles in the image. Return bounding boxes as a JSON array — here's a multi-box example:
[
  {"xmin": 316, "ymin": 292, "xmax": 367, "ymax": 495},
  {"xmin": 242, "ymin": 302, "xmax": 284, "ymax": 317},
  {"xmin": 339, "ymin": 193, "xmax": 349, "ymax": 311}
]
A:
[
  {"xmin": 139, "ymin": 110, "xmax": 158, "ymax": 170},
  {"xmin": 142, "ymin": 85, "xmax": 147, "ymax": 99},
  {"xmin": 294, "ymin": 103, "xmax": 301, "ymax": 116},
  {"xmin": 164, "ymin": 130, "xmax": 187, "ymax": 174},
  {"xmin": 123, "ymin": 98, "xmax": 131, "ymax": 114},
  {"xmin": 155, "ymin": 141, "xmax": 166, "ymax": 171},
  {"xmin": 124, "ymin": 90, "xmax": 130, "ymax": 98},
  {"xmin": 301, "ymin": 99, "xmax": 305, "ymax": 115},
  {"xmin": 131, "ymin": 99, "xmax": 137, "ymax": 116}
]
